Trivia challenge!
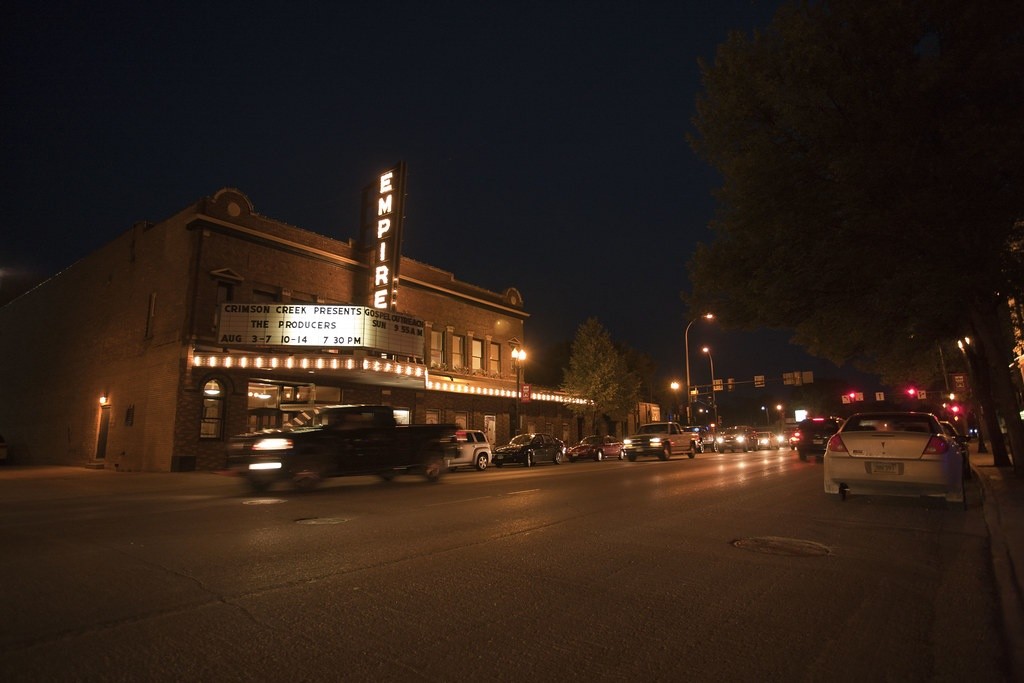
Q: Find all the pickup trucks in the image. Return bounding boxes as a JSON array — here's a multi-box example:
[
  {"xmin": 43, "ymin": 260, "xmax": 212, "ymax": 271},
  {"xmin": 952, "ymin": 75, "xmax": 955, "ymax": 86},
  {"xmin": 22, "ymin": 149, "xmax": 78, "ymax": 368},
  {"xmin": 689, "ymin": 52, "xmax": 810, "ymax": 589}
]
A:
[
  {"xmin": 228, "ymin": 403, "xmax": 462, "ymax": 495},
  {"xmin": 620, "ymin": 421, "xmax": 699, "ymax": 461}
]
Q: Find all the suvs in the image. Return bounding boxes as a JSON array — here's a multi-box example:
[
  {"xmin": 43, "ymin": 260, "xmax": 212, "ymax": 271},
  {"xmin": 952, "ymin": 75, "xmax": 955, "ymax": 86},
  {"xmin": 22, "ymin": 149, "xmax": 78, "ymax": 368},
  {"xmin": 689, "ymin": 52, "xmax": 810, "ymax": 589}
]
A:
[
  {"xmin": 798, "ymin": 416, "xmax": 846, "ymax": 461},
  {"xmin": 446, "ymin": 429, "xmax": 492, "ymax": 472},
  {"xmin": 718, "ymin": 426, "xmax": 758, "ymax": 454}
]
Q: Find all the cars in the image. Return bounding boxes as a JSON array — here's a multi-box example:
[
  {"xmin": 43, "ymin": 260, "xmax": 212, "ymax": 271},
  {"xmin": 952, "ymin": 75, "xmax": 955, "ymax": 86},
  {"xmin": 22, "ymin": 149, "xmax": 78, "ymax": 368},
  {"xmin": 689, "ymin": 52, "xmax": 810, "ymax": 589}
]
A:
[
  {"xmin": 683, "ymin": 426, "xmax": 725, "ymax": 454},
  {"xmin": 790, "ymin": 431, "xmax": 801, "ymax": 450},
  {"xmin": 492, "ymin": 432, "xmax": 566, "ymax": 467},
  {"xmin": 940, "ymin": 420, "xmax": 970, "ymax": 477},
  {"xmin": 823, "ymin": 409, "xmax": 970, "ymax": 506},
  {"xmin": 565, "ymin": 434, "xmax": 625, "ymax": 462},
  {"xmin": 757, "ymin": 431, "xmax": 780, "ymax": 450}
]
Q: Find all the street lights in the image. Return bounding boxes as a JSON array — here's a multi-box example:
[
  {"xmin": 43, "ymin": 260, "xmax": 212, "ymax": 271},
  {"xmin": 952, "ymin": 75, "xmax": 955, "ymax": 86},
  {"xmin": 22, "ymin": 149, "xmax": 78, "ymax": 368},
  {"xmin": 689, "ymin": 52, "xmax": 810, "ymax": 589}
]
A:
[
  {"xmin": 685, "ymin": 314, "xmax": 713, "ymax": 425},
  {"xmin": 511, "ymin": 347, "xmax": 527, "ymax": 434},
  {"xmin": 702, "ymin": 348, "xmax": 717, "ymax": 427}
]
